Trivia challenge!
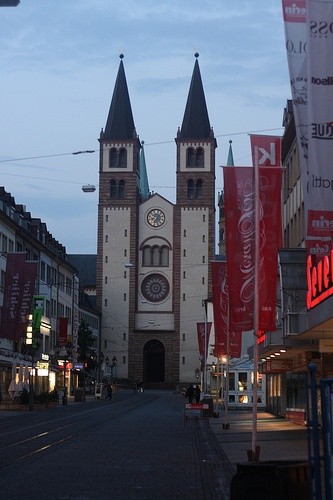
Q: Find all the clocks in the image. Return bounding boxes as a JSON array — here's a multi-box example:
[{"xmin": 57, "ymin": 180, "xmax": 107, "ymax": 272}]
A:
[{"xmin": 147, "ymin": 209, "xmax": 166, "ymax": 227}]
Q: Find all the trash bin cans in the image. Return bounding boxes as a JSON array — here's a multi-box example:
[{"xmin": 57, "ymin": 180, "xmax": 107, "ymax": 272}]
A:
[
  {"xmin": 74, "ymin": 388, "xmax": 85, "ymax": 402},
  {"xmin": 200, "ymin": 395, "xmax": 214, "ymax": 418}
]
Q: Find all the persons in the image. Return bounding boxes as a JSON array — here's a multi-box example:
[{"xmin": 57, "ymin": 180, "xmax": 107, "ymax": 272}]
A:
[
  {"xmin": 105, "ymin": 382, "xmax": 113, "ymax": 401},
  {"xmin": 133, "ymin": 380, "xmax": 144, "ymax": 395},
  {"xmin": 184, "ymin": 382, "xmax": 201, "ymax": 402}
]
